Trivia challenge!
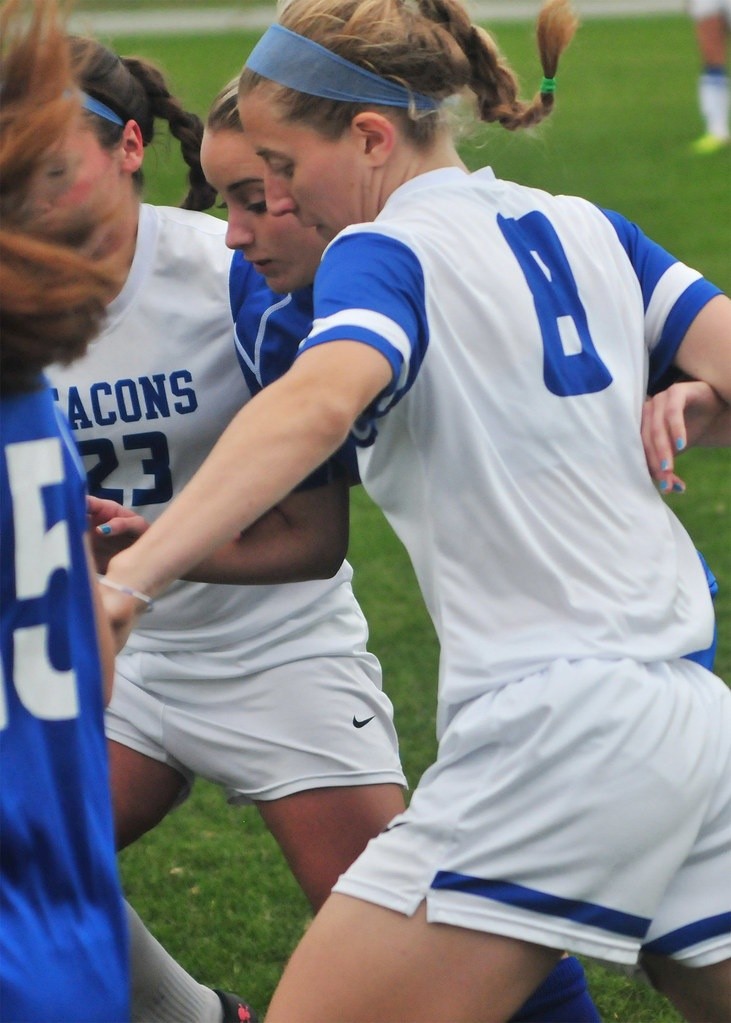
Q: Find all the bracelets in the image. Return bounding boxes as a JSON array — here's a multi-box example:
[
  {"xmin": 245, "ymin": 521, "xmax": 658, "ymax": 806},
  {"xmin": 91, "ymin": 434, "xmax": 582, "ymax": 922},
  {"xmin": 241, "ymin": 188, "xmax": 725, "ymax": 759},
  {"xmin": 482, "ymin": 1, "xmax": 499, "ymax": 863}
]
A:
[{"xmin": 98, "ymin": 577, "xmax": 154, "ymax": 612}]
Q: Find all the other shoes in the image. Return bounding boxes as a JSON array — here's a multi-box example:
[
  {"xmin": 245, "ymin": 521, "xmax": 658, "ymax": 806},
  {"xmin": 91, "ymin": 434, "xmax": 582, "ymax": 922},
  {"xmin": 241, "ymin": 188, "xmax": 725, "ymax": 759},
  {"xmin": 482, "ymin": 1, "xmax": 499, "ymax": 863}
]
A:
[
  {"xmin": 214, "ymin": 990, "xmax": 260, "ymax": 1023},
  {"xmin": 692, "ymin": 131, "xmax": 725, "ymax": 155}
]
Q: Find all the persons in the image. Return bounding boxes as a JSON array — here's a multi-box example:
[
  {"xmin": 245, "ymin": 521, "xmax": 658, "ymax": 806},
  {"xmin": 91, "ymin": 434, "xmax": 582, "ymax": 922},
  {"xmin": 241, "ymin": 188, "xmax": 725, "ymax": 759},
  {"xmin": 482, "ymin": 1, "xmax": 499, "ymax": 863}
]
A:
[
  {"xmin": 97, "ymin": 0, "xmax": 731, "ymax": 1022},
  {"xmin": 80, "ymin": 74, "xmax": 731, "ymax": 672},
  {"xmin": 0, "ymin": 2, "xmax": 132, "ymax": 1022},
  {"xmin": 685, "ymin": 2, "xmax": 731, "ymax": 153},
  {"xmin": 30, "ymin": 34, "xmax": 603, "ymax": 1022}
]
